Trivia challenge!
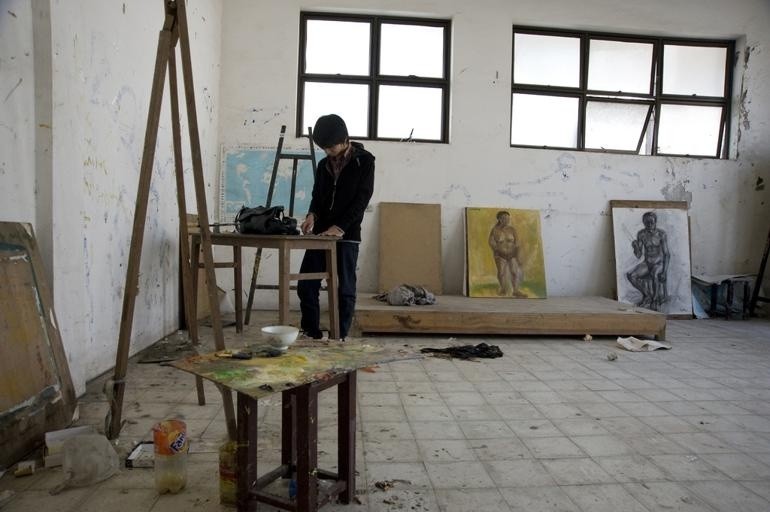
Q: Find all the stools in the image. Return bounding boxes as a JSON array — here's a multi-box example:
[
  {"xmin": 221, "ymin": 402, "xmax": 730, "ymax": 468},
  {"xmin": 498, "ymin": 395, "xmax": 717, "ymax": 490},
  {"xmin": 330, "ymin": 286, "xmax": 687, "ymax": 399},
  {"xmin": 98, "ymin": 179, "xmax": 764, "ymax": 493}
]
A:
[
  {"xmin": 710, "ymin": 279, "xmax": 750, "ymax": 321},
  {"xmin": 237, "ymin": 367, "xmax": 357, "ymax": 510}
]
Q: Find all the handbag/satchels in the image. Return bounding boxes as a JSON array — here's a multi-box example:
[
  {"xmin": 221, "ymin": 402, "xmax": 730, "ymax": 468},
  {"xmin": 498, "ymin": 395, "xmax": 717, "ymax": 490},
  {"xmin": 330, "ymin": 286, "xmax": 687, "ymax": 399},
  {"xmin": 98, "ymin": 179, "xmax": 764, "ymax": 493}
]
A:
[{"xmin": 232, "ymin": 204, "xmax": 300, "ymax": 235}]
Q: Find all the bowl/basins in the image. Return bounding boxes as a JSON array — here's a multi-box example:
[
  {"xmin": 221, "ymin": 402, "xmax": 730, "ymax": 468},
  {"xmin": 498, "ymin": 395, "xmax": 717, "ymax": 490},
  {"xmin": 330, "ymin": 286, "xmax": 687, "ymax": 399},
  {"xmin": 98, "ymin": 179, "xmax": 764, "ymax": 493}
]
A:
[{"xmin": 261, "ymin": 325, "xmax": 300, "ymax": 351}]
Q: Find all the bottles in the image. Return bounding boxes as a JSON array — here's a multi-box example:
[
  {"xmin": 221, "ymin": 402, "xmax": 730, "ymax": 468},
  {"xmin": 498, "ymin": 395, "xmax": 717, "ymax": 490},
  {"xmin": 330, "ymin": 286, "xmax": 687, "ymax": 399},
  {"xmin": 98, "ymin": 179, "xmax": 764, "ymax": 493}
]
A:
[
  {"xmin": 218, "ymin": 441, "xmax": 239, "ymax": 506},
  {"xmin": 152, "ymin": 419, "xmax": 189, "ymax": 496}
]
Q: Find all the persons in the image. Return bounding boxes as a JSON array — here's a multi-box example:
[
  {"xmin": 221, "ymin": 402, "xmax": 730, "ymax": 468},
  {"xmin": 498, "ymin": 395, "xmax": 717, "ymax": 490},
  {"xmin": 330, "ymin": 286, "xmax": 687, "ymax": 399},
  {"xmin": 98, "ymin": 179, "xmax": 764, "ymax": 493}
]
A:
[
  {"xmin": 488, "ymin": 211, "xmax": 529, "ymax": 296},
  {"xmin": 297, "ymin": 114, "xmax": 375, "ymax": 342},
  {"xmin": 628, "ymin": 212, "xmax": 670, "ymax": 311}
]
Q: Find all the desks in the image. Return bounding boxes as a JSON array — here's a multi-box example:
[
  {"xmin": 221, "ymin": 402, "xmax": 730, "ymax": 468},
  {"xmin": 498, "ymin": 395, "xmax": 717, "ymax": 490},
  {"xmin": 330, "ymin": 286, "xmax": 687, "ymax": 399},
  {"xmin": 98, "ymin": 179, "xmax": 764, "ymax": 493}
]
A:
[{"xmin": 185, "ymin": 232, "xmax": 340, "ymax": 339}]
